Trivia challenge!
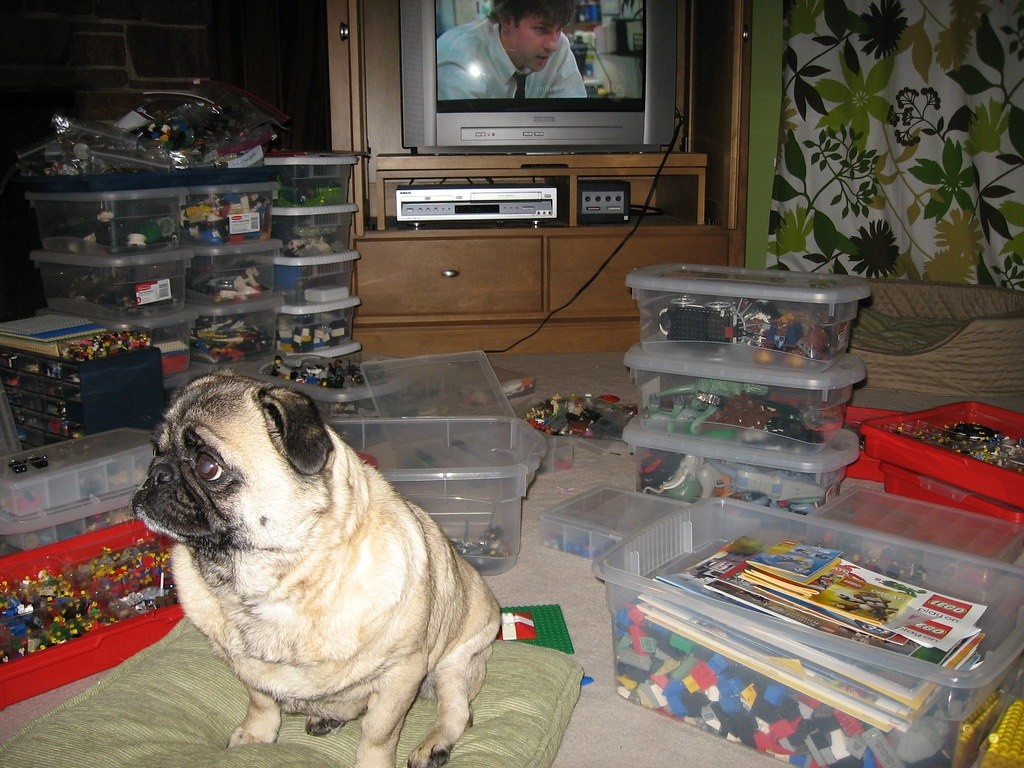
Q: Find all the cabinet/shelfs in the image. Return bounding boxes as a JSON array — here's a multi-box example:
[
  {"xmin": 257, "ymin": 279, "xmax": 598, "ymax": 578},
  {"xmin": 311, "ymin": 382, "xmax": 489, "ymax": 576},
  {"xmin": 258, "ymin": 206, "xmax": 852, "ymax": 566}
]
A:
[{"xmin": 327, "ymin": 0, "xmax": 753, "ymax": 353}]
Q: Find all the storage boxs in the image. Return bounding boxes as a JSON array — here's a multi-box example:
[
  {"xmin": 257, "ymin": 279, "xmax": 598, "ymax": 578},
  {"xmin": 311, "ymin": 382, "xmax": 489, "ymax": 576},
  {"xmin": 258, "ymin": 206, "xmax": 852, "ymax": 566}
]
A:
[{"xmin": 0, "ymin": 150, "xmax": 1024, "ymax": 768}]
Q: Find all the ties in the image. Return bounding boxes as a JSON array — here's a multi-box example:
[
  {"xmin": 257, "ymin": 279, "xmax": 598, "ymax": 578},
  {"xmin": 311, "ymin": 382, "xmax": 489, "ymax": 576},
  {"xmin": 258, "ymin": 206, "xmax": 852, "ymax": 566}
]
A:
[{"xmin": 513, "ymin": 73, "xmax": 527, "ymax": 99}]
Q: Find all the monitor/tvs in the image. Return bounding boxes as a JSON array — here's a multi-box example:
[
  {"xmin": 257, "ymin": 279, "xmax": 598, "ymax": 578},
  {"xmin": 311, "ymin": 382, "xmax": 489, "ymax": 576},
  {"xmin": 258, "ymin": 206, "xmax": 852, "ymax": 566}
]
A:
[{"xmin": 399, "ymin": 0, "xmax": 679, "ymax": 154}]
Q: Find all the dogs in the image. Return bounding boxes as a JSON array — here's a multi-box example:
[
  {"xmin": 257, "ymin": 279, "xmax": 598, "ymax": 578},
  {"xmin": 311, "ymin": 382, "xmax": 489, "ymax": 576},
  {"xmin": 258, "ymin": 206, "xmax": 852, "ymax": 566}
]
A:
[{"xmin": 131, "ymin": 366, "xmax": 502, "ymax": 768}]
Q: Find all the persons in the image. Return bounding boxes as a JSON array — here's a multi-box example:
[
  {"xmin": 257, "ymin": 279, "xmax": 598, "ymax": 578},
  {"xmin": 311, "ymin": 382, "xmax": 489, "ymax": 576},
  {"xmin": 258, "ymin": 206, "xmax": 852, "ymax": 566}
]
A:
[{"xmin": 436, "ymin": 0, "xmax": 589, "ymax": 101}]
